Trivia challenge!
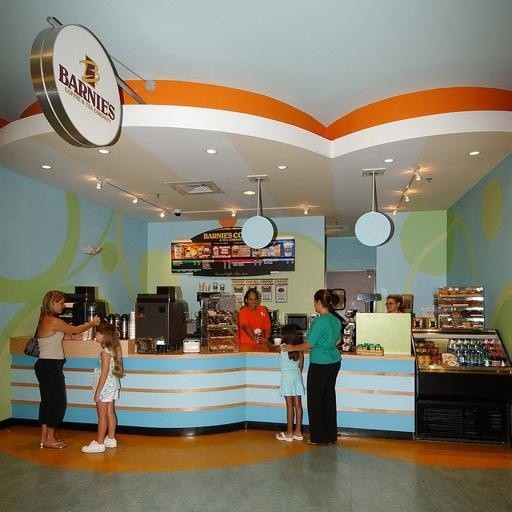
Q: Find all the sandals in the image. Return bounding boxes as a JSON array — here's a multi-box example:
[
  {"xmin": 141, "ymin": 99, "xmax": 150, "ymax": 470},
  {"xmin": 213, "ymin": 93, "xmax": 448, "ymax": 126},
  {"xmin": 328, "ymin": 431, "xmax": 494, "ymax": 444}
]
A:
[{"xmin": 39, "ymin": 437, "xmax": 69, "ymax": 450}]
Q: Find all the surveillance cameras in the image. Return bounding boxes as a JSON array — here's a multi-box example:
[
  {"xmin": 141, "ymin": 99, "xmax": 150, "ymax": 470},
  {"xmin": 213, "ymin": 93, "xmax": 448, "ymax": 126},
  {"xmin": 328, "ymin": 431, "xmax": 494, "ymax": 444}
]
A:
[{"xmin": 174, "ymin": 210, "xmax": 182, "ymax": 216}]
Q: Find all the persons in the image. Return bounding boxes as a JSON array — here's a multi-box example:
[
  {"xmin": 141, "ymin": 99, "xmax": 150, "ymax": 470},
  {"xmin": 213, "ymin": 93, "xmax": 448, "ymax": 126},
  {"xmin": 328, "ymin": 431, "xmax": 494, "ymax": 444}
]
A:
[
  {"xmin": 282, "ymin": 289, "xmax": 342, "ymax": 446},
  {"xmin": 34, "ymin": 291, "xmax": 101, "ymax": 449},
  {"xmin": 259, "ymin": 324, "xmax": 305, "ymax": 442},
  {"xmin": 385, "ymin": 295, "xmax": 403, "ymax": 313},
  {"xmin": 81, "ymin": 324, "xmax": 126, "ymax": 453},
  {"xmin": 238, "ymin": 289, "xmax": 271, "ymax": 344}
]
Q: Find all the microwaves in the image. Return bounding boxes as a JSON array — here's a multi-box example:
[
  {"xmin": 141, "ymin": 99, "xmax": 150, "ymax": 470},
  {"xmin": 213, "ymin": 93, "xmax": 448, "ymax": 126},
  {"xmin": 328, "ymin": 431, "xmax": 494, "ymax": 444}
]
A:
[{"xmin": 285, "ymin": 314, "xmax": 317, "ymax": 333}]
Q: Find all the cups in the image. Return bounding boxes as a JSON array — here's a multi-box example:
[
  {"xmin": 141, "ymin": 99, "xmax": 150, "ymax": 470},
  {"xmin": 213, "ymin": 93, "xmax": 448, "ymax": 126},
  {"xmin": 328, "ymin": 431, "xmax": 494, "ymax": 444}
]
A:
[
  {"xmin": 87, "ymin": 306, "xmax": 135, "ymax": 340},
  {"xmin": 273, "ymin": 338, "xmax": 282, "ymax": 346}
]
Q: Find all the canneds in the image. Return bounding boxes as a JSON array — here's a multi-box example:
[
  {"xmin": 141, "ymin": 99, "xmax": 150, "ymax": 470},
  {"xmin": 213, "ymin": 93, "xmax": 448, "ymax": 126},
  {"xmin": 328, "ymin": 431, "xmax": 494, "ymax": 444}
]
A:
[{"xmin": 415, "ymin": 338, "xmax": 506, "ymax": 367}]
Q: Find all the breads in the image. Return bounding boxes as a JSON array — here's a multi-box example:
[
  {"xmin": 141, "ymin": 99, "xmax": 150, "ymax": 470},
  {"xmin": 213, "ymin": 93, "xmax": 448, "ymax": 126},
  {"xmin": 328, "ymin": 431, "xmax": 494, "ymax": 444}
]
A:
[{"xmin": 438, "ymin": 290, "xmax": 484, "ymax": 328}]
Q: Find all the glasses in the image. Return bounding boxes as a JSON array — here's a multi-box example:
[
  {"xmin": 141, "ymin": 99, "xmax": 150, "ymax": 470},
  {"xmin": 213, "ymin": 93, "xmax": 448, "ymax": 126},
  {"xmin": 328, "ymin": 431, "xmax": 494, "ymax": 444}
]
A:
[{"xmin": 384, "ymin": 301, "xmax": 399, "ymax": 306}]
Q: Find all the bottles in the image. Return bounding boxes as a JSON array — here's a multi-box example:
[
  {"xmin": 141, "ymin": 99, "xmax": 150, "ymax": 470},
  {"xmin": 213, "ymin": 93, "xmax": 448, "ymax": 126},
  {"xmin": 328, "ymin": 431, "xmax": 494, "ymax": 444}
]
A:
[
  {"xmin": 447, "ymin": 336, "xmax": 508, "ymax": 368},
  {"xmin": 212, "ymin": 282, "xmax": 226, "ymax": 293}
]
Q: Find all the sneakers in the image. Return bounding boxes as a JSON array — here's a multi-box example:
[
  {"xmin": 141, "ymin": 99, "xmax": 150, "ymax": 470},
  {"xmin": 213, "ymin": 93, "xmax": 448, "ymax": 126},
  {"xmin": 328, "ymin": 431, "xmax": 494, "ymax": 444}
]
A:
[
  {"xmin": 102, "ymin": 435, "xmax": 120, "ymax": 450},
  {"xmin": 81, "ymin": 439, "xmax": 107, "ymax": 454}
]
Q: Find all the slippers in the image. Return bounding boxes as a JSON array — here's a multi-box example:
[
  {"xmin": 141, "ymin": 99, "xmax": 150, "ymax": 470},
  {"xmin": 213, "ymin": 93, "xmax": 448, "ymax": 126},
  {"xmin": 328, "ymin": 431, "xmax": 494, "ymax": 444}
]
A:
[
  {"xmin": 275, "ymin": 430, "xmax": 295, "ymax": 443},
  {"xmin": 291, "ymin": 431, "xmax": 305, "ymax": 442}
]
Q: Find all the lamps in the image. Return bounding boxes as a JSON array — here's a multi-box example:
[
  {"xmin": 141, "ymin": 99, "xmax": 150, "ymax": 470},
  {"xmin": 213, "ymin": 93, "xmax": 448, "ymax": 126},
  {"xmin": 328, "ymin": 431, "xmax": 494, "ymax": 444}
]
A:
[
  {"xmin": 355, "ymin": 167, "xmax": 395, "ymax": 247},
  {"xmin": 95, "ymin": 176, "xmax": 170, "ymax": 217},
  {"xmin": 393, "ymin": 163, "xmax": 422, "ymax": 216},
  {"xmin": 181, "ymin": 208, "xmax": 320, "ymax": 217},
  {"xmin": 242, "ymin": 175, "xmax": 278, "ymax": 249}
]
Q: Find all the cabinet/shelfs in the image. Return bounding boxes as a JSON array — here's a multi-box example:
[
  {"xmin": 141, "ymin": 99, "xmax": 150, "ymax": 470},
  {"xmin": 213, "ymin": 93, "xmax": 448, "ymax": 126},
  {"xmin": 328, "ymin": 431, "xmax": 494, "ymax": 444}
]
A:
[{"xmin": 433, "ymin": 287, "xmax": 485, "ymax": 327}]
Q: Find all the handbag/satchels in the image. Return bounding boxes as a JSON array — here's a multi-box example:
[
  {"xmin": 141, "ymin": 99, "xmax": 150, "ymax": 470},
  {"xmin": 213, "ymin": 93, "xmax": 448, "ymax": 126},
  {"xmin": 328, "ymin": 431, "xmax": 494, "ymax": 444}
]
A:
[{"xmin": 24, "ymin": 336, "xmax": 40, "ymax": 359}]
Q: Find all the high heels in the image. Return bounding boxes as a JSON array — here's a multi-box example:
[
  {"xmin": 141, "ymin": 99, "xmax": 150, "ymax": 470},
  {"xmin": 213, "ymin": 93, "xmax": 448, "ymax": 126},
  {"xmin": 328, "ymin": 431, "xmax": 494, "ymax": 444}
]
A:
[{"xmin": 305, "ymin": 436, "xmax": 338, "ymax": 446}]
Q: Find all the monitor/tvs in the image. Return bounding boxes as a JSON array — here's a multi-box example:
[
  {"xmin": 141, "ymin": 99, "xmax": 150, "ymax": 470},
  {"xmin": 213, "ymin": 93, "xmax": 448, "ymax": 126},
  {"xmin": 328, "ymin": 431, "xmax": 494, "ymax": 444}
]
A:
[{"xmin": 286, "ymin": 313, "xmax": 309, "ymax": 334}]
